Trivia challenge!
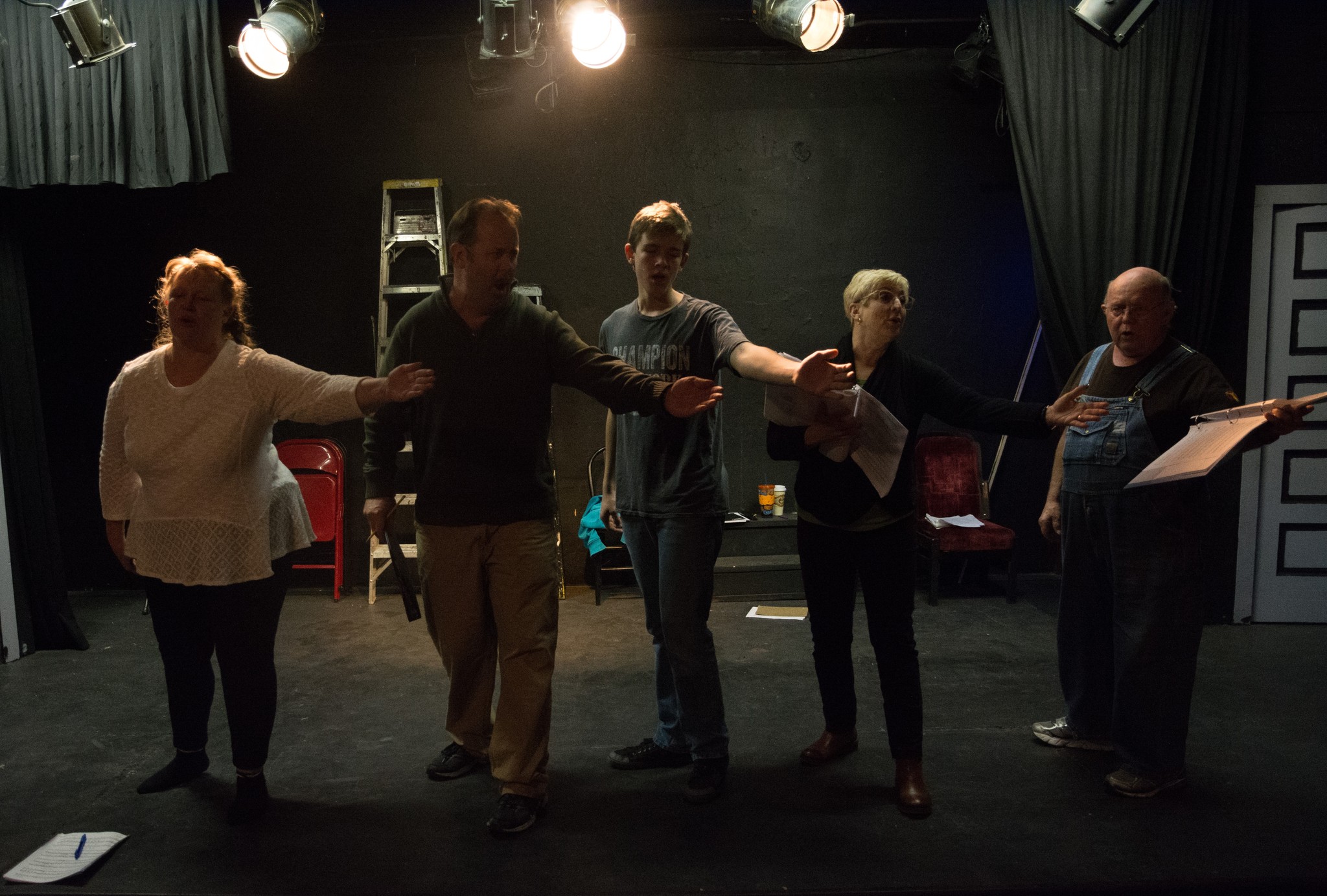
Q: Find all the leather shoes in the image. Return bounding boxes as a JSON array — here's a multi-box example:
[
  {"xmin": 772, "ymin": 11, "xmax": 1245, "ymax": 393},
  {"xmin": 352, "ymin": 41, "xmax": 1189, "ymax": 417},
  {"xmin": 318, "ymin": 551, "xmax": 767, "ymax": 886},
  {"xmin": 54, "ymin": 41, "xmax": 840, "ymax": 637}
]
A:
[
  {"xmin": 893, "ymin": 759, "xmax": 933, "ymax": 817},
  {"xmin": 800, "ymin": 728, "xmax": 860, "ymax": 767}
]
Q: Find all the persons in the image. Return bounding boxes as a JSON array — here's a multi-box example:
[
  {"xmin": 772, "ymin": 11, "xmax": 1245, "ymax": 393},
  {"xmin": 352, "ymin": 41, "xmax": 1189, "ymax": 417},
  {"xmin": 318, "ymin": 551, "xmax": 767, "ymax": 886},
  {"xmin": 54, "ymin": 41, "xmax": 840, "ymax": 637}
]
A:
[
  {"xmin": 761, "ymin": 268, "xmax": 1110, "ymax": 818},
  {"xmin": 584, "ymin": 199, "xmax": 859, "ymax": 799},
  {"xmin": 361, "ymin": 192, "xmax": 728, "ymax": 838},
  {"xmin": 1017, "ymin": 262, "xmax": 1313, "ymax": 802},
  {"xmin": 92, "ymin": 247, "xmax": 439, "ymax": 810}
]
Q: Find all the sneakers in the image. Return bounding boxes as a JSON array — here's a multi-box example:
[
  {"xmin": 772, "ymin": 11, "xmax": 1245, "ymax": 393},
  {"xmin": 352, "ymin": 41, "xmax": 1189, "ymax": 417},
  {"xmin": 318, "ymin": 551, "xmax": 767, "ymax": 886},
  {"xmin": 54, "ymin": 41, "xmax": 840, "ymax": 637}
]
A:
[
  {"xmin": 1106, "ymin": 764, "xmax": 1192, "ymax": 797},
  {"xmin": 485, "ymin": 793, "xmax": 549, "ymax": 833},
  {"xmin": 426, "ymin": 740, "xmax": 477, "ymax": 778},
  {"xmin": 687, "ymin": 754, "xmax": 732, "ymax": 796},
  {"xmin": 608, "ymin": 738, "xmax": 688, "ymax": 768},
  {"xmin": 1033, "ymin": 716, "xmax": 1118, "ymax": 752}
]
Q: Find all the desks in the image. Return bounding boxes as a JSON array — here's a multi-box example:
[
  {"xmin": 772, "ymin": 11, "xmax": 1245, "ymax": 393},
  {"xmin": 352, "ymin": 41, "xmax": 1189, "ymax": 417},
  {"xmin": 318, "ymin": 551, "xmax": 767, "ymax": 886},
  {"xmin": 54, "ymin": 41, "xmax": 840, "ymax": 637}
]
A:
[{"xmin": 707, "ymin": 510, "xmax": 819, "ymax": 606}]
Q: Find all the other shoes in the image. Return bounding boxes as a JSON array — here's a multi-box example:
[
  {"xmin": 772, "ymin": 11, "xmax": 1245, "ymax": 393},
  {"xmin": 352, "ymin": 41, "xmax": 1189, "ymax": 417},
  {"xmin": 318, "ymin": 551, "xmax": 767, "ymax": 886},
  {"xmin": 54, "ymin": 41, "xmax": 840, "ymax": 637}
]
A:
[
  {"xmin": 135, "ymin": 749, "xmax": 213, "ymax": 794},
  {"xmin": 234, "ymin": 770, "xmax": 270, "ymax": 804}
]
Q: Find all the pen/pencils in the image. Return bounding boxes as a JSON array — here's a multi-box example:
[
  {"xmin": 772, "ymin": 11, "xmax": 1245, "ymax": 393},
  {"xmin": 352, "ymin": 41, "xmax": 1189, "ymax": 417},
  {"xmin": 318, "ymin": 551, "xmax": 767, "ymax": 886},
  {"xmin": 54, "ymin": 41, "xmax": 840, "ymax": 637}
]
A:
[
  {"xmin": 778, "ymin": 515, "xmax": 788, "ymax": 520},
  {"xmin": 75, "ymin": 833, "xmax": 87, "ymax": 860}
]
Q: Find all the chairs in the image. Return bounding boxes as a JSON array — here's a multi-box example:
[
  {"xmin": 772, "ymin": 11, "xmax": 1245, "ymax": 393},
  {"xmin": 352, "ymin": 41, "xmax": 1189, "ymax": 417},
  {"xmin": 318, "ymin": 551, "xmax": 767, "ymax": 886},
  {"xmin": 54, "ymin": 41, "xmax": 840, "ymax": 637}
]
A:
[
  {"xmin": 267, "ymin": 438, "xmax": 355, "ymax": 603},
  {"xmin": 913, "ymin": 431, "xmax": 1022, "ymax": 607},
  {"xmin": 589, "ymin": 444, "xmax": 655, "ymax": 606}
]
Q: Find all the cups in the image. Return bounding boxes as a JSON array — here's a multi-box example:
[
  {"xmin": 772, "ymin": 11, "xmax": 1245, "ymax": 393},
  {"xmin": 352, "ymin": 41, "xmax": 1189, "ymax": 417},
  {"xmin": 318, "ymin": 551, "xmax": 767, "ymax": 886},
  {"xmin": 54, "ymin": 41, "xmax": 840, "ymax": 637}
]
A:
[{"xmin": 758, "ymin": 484, "xmax": 786, "ymax": 518}]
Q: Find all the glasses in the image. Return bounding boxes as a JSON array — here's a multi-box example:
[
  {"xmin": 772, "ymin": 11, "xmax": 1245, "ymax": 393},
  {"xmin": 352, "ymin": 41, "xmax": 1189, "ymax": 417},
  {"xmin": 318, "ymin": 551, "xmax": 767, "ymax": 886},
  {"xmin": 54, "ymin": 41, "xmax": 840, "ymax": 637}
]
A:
[{"xmin": 857, "ymin": 290, "xmax": 916, "ymax": 311}]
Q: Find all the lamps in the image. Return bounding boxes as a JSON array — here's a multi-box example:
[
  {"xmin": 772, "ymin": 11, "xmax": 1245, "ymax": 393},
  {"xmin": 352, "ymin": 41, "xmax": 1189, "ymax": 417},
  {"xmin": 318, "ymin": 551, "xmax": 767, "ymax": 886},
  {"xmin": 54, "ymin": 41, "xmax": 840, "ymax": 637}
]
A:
[
  {"xmin": 18, "ymin": 0, "xmax": 142, "ymax": 71},
  {"xmin": 228, "ymin": 0, "xmax": 327, "ymax": 83},
  {"xmin": 544, "ymin": 1, "xmax": 636, "ymax": 74},
  {"xmin": 1066, "ymin": 0, "xmax": 1162, "ymax": 49},
  {"xmin": 747, "ymin": 0, "xmax": 858, "ymax": 55},
  {"xmin": 469, "ymin": 0, "xmax": 550, "ymax": 72}
]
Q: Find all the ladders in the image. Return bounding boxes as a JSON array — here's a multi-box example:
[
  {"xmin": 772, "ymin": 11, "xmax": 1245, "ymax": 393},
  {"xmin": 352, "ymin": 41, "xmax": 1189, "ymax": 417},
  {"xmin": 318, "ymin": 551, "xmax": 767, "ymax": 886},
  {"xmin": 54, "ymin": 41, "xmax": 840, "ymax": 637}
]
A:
[
  {"xmin": 359, "ymin": 176, "xmax": 450, "ymax": 604},
  {"xmin": 507, "ymin": 282, "xmax": 569, "ymax": 604}
]
